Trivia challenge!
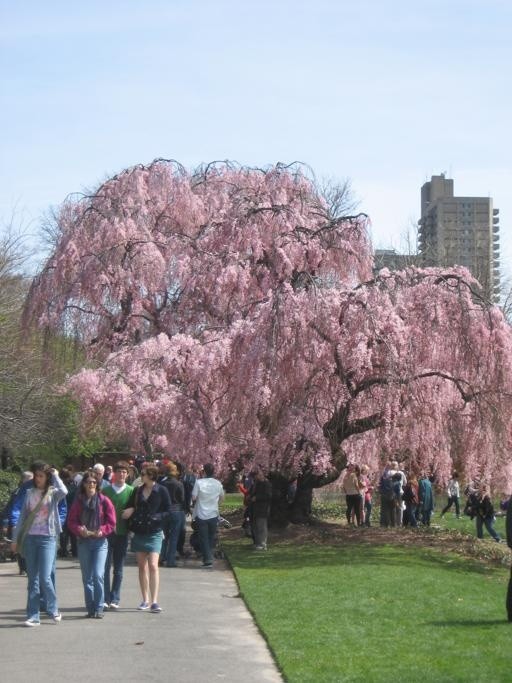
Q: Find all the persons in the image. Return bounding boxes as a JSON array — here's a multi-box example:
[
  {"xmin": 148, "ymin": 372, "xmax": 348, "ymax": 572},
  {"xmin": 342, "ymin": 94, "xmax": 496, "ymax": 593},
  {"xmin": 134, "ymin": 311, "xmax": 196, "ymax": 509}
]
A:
[
  {"xmin": 237, "ymin": 469, "xmax": 271, "ymax": 550},
  {"xmin": 441, "ymin": 472, "xmax": 462, "ymax": 519},
  {"xmin": 97, "ymin": 460, "xmax": 135, "ymax": 613},
  {"xmin": 6, "ymin": 455, "xmax": 232, "ymax": 575},
  {"xmin": 10, "ymin": 461, "xmax": 69, "ymax": 626},
  {"xmin": 125, "ymin": 464, "xmax": 167, "ymax": 614},
  {"xmin": 64, "ymin": 471, "xmax": 118, "ymax": 618},
  {"xmin": 343, "ymin": 465, "xmax": 373, "ymax": 528},
  {"xmin": 379, "ymin": 462, "xmax": 434, "ymax": 527},
  {"xmin": 464, "ymin": 483, "xmax": 500, "ymax": 542}
]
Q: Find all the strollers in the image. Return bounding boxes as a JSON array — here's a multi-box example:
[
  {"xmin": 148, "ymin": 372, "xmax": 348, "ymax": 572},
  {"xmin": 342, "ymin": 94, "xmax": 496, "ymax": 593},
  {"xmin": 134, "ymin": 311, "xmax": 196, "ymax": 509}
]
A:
[{"xmin": 186, "ymin": 516, "xmax": 225, "ymax": 560}]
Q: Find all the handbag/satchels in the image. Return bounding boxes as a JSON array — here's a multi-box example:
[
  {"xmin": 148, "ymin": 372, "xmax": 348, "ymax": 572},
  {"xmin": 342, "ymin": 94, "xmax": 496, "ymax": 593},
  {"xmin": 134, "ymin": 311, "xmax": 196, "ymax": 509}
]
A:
[{"xmin": 16, "ymin": 512, "xmax": 34, "ymax": 554}]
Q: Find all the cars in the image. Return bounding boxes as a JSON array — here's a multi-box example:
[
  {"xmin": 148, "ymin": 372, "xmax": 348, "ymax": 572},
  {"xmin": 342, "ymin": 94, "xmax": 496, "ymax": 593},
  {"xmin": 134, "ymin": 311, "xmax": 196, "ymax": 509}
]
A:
[{"xmin": 93, "ymin": 452, "xmax": 163, "ymax": 467}]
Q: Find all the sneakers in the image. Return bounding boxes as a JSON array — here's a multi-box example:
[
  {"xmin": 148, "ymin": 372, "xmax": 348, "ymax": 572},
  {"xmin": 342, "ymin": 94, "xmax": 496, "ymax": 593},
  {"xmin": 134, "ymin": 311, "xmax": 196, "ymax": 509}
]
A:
[
  {"xmin": 151, "ymin": 603, "xmax": 162, "ymax": 613},
  {"xmin": 25, "ymin": 610, "xmax": 62, "ymax": 626},
  {"xmin": 138, "ymin": 601, "xmax": 149, "ymax": 612},
  {"xmin": 86, "ymin": 601, "xmax": 120, "ymax": 619}
]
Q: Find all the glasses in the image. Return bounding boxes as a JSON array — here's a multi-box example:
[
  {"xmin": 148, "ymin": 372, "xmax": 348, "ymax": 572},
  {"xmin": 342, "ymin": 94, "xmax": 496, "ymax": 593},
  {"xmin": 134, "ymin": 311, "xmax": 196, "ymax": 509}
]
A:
[{"xmin": 84, "ymin": 480, "xmax": 98, "ymax": 485}]
